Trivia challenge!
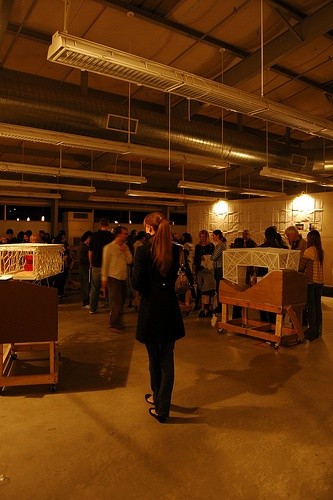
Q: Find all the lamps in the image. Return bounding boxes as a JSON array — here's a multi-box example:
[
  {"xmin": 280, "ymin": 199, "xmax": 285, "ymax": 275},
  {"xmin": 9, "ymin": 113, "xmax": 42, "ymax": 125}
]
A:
[{"xmin": 0, "ymin": 0, "xmax": 333, "ymax": 206}]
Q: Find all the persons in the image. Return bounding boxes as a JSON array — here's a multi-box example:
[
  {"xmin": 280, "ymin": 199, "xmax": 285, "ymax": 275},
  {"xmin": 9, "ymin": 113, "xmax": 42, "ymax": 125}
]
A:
[
  {"xmin": 0, "ymin": 221, "xmax": 323, "ymax": 343},
  {"xmin": 130, "ymin": 213, "xmax": 186, "ymax": 422}
]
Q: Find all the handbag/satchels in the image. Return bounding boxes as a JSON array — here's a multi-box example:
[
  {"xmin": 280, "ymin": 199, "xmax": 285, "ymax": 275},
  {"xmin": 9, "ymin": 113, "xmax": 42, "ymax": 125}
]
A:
[{"xmin": 175, "ymin": 246, "xmax": 189, "ymax": 294}]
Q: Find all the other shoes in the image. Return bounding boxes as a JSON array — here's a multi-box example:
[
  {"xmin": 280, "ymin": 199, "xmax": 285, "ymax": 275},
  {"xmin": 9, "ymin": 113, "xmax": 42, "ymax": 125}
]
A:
[
  {"xmin": 62, "ymin": 295, "xmax": 67, "ymax": 297},
  {"xmin": 214, "ymin": 309, "xmax": 221, "ymax": 314},
  {"xmin": 206, "ymin": 310, "xmax": 213, "ymax": 317},
  {"xmin": 89, "ymin": 310, "xmax": 95, "ymax": 313},
  {"xmin": 82, "ymin": 304, "xmax": 90, "ymax": 309},
  {"xmin": 303, "ymin": 328, "xmax": 320, "ymax": 343},
  {"xmin": 198, "ymin": 311, "xmax": 206, "ymax": 318},
  {"xmin": 145, "ymin": 394, "xmax": 155, "ymax": 405},
  {"xmin": 194, "ymin": 306, "xmax": 200, "ymax": 311},
  {"xmin": 68, "ymin": 280, "xmax": 72, "ymax": 285},
  {"xmin": 109, "ymin": 325, "xmax": 129, "ymax": 334},
  {"xmin": 58, "ymin": 296, "xmax": 63, "ymax": 298},
  {"xmin": 128, "ymin": 304, "xmax": 135, "ymax": 308},
  {"xmin": 149, "ymin": 408, "xmax": 167, "ymax": 423},
  {"xmin": 289, "ymin": 318, "xmax": 309, "ymax": 328}
]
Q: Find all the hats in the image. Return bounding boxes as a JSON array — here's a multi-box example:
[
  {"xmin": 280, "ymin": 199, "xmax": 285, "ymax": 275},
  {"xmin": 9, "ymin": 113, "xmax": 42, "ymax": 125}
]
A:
[{"xmin": 243, "ymin": 230, "xmax": 250, "ymax": 234}]
